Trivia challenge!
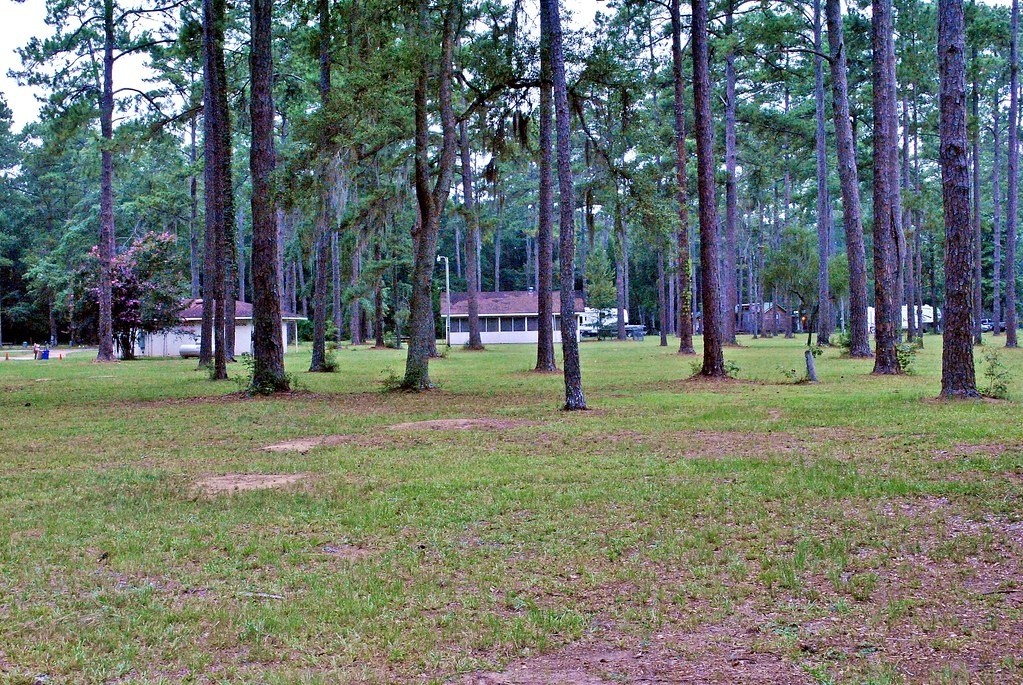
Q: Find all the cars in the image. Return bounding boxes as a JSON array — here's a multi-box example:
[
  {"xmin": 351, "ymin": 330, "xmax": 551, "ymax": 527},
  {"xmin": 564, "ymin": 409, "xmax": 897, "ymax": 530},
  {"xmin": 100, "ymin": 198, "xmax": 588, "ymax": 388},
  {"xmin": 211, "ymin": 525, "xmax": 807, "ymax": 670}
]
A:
[{"xmin": 981, "ymin": 318, "xmax": 1008, "ymax": 332}]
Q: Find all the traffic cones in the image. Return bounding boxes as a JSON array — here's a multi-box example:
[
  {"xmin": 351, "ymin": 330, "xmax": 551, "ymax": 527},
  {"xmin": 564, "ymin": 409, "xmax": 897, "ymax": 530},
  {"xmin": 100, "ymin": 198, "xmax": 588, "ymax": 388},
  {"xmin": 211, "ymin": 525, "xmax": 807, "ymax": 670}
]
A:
[
  {"xmin": 59, "ymin": 354, "xmax": 62, "ymax": 360},
  {"xmin": 5, "ymin": 353, "xmax": 8, "ymax": 359}
]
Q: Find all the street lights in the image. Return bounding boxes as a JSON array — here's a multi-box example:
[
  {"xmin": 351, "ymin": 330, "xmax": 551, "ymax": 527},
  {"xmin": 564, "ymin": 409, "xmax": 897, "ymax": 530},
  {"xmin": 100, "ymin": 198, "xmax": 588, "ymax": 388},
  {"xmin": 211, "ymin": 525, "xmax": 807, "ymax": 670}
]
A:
[{"xmin": 437, "ymin": 255, "xmax": 450, "ymax": 348}]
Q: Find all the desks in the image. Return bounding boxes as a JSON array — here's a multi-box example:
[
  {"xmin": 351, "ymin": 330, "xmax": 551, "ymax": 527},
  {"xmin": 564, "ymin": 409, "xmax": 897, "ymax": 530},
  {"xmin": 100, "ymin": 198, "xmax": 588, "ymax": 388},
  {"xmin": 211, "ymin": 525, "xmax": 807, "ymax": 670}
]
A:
[
  {"xmin": 600, "ymin": 326, "xmax": 644, "ymax": 341},
  {"xmin": 1, "ymin": 343, "xmax": 13, "ymax": 350}
]
